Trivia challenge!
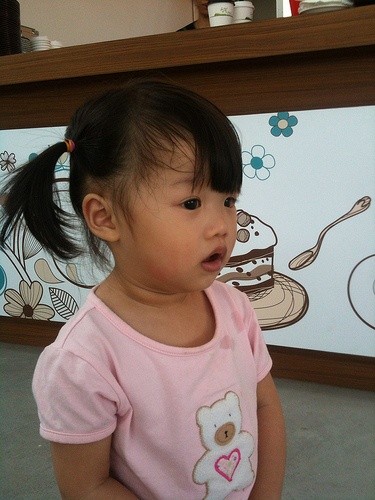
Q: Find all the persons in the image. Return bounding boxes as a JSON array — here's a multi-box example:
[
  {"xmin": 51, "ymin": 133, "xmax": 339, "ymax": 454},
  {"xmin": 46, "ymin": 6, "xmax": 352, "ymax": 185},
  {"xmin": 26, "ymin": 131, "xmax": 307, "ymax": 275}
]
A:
[{"xmin": 0, "ymin": 78, "xmax": 293, "ymax": 499}]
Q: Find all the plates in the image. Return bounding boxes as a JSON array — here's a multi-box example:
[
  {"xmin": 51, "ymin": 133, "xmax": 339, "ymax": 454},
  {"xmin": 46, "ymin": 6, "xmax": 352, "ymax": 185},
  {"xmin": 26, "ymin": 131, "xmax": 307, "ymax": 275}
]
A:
[{"xmin": 299, "ymin": 5, "xmax": 346, "ymax": 15}]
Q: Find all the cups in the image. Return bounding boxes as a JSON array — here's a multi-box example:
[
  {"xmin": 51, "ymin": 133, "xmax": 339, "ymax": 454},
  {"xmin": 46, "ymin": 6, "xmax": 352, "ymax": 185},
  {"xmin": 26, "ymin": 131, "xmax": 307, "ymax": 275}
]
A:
[
  {"xmin": 233, "ymin": 2, "xmax": 255, "ymax": 25},
  {"xmin": 205, "ymin": 0, "xmax": 236, "ymax": 28}
]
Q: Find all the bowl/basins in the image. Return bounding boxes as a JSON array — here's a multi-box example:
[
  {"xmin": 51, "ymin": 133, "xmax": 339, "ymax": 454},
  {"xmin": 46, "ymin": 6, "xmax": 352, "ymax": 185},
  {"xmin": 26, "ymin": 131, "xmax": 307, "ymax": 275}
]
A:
[{"xmin": 31, "ymin": 36, "xmax": 62, "ymax": 52}]
